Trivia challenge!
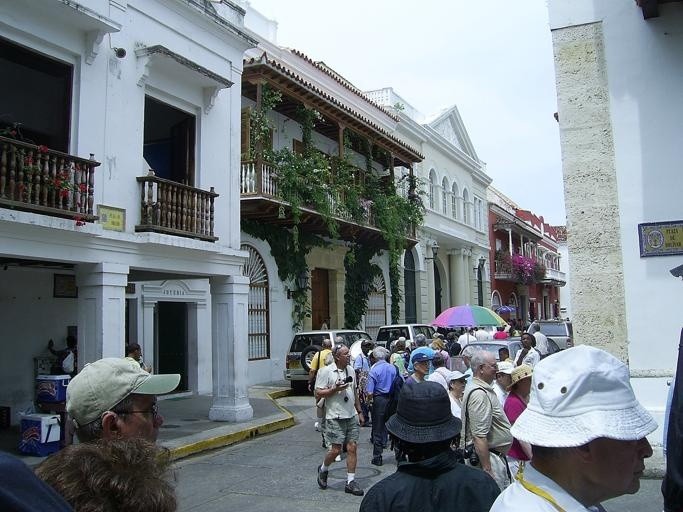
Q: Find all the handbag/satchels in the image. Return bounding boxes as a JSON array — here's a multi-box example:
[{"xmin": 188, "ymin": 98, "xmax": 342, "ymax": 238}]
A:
[{"xmin": 310, "ymin": 377, "xmax": 316, "ymax": 391}]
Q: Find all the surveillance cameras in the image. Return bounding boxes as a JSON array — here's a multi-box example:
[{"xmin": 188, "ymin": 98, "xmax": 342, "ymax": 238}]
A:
[{"xmin": 112, "ymin": 47, "xmax": 127, "ymax": 59}]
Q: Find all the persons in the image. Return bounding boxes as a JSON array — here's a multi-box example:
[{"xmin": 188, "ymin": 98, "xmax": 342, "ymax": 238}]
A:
[
  {"xmin": 0, "ymin": 327, "xmax": 184, "ymax": 511},
  {"xmin": 307, "ymin": 317, "xmax": 683, "ymax": 512}
]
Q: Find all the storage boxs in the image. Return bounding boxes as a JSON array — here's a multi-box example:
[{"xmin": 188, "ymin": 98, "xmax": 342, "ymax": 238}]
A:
[
  {"xmin": 14, "ymin": 410, "xmax": 61, "ymax": 459},
  {"xmin": 33, "ymin": 371, "xmax": 71, "ymax": 402}
]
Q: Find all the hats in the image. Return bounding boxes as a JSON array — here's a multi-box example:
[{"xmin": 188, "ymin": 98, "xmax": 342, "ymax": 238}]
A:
[
  {"xmin": 496, "ymin": 355, "xmax": 551, "ymax": 448},
  {"xmin": 65, "ymin": 356, "xmax": 181, "ymax": 430},
  {"xmin": 385, "ymin": 353, "xmax": 470, "ymax": 444}
]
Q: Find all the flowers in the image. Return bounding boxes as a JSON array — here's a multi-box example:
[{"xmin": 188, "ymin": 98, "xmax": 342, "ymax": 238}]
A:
[{"xmin": 0, "ymin": 124, "xmax": 90, "ymax": 228}]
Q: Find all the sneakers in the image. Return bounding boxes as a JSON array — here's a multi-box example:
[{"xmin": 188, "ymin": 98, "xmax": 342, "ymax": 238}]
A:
[
  {"xmin": 317, "ymin": 464, "xmax": 329, "ymax": 489},
  {"xmin": 344, "ymin": 480, "xmax": 364, "ymax": 496}
]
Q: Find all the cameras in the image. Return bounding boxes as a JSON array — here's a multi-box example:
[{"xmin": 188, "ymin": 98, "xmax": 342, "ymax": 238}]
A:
[
  {"xmin": 464, "ymin": 444, "xmax": 479, "ymax": 466},
  {"xmin": 343, "ymin": 376, "xmax": 353, "ymax": 384}
]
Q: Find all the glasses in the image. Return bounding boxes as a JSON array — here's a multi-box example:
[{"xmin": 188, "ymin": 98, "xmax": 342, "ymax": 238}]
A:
[{"xmin": 110, "ymin": 401, "xmax": 159, "ymax": 421}]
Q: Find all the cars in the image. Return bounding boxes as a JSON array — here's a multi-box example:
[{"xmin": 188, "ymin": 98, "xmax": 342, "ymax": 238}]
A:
[
  {"xmin": 457, "ymin": 340, "xmax": 540, "ymax": 363},
  {"xmin": 503, "ymin": 335, "xmax": 565, "ymax": 358}
]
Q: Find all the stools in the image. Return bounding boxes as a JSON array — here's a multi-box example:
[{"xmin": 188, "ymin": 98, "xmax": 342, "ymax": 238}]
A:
[{"xmin": 34, "ymin": 397, "xmax": 67, "ymax": 441}]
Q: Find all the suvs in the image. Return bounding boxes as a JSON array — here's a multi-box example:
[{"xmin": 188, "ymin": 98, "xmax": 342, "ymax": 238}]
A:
[
  {"xmin": 526, "ymin": 319, "xmax": 573, "ymax": 351},
  {"xmin": 282, "ymin": 322, "xmax": 377, "ymax": 393}
]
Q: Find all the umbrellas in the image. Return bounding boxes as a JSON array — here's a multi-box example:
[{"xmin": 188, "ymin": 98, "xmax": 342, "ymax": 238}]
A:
[{"xmin": 429, "ymin": 305, "xmax": 506, "ymax": 345}]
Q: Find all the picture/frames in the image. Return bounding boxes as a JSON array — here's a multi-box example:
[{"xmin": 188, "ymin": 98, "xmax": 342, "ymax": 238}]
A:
[{"xmin": 49, "ymin": 270, "xmax": 78, "ymax": 299}]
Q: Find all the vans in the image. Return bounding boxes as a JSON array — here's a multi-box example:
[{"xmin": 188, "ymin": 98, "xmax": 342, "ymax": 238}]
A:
[{"xmin": 374, "ymin": 323, "xmax": 436, "ymax": 351}]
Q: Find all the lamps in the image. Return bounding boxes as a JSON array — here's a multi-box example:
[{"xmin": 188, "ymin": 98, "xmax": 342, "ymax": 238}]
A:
[
  {"xmin": 284, "ymin": 266, "xmax": 310, "ymax": 301},
  {"xmin": 421, "ymin": 239, "xmax": 440, "ymax": 261},
  {"xmin": 356, "ymin": 277, "xmax": 369, "ymax": 301},
  {"xmin": 505, "ymin": 294, "xmax": 515, "ymax": 307},
  {"xmin": 471, "ymin": 255, "xmax": 486, "ymax": 271}
]
